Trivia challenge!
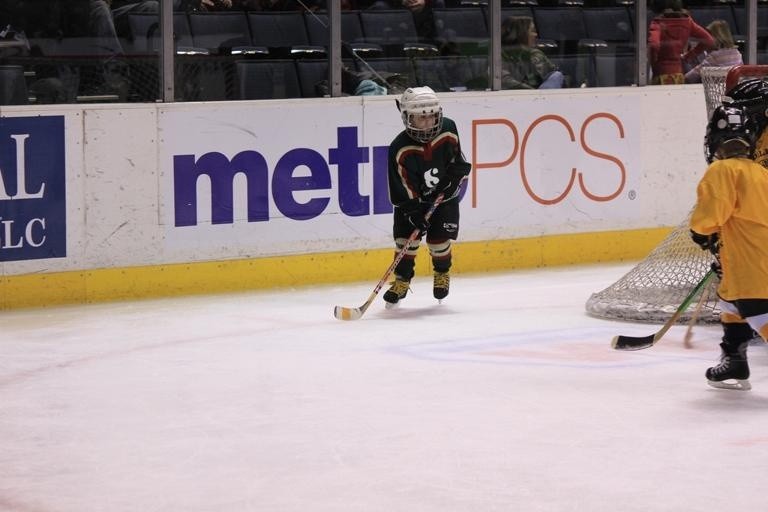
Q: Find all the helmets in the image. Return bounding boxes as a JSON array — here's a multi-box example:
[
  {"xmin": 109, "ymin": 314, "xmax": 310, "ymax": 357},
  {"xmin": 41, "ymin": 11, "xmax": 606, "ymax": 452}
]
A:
[
  {"xmin": 399, "ymin": 85, "xmax": 443, "ymax": 120},
  {"xmin": 706, "ymin": 78, "xmax": 768, "ymax": 153}
]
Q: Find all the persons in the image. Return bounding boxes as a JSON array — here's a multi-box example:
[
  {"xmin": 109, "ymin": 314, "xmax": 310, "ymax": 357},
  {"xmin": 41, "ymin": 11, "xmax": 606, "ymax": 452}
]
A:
[
  {"xmin": 382, "ymin": 85, "xmax": 472, "ymax": 303},
  {"xmin": 688, "ymin": 78, "xmax": 768, "ymax": 381},
  {"xmin": 2, "ymin": 1, "xmax": 743, "ymax": 103}
]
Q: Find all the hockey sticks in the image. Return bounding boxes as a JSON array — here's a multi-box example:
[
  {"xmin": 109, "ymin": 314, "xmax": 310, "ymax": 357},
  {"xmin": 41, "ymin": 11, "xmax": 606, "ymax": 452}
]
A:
[
  {"xmin": 608, "ymin": 268, "xmax": 717, "ymax": 350},
  {"xmin": 334, "ymin": 195, "xmax": 445, "ymax": 320},
  {"xmin": 683, "ymin": 274, "xmax": 714, "ymax": 341}
]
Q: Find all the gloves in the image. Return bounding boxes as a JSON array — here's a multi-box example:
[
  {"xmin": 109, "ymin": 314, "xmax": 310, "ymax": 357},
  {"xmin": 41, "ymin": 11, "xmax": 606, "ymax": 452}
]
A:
[
  {"xmin": 710, "ymin": 261, "xmax": 725, "ymax": 280},
  {"xmin": 397, "ymin": 198, "xmax": 431, "ymax": 237},
  {"xmin": 439, "ymin": 168, "xmax": 465, "ymax": 202},
  {"xmin": 689, "ymin": 229, "xmax": 719, "ymax": 255}
]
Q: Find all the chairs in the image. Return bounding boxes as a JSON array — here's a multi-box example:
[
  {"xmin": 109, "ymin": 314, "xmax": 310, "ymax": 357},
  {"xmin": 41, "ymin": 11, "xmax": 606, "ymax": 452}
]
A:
[{"xmin": 2, "ymin": 1, "xmax": 768, "ymax": 103}]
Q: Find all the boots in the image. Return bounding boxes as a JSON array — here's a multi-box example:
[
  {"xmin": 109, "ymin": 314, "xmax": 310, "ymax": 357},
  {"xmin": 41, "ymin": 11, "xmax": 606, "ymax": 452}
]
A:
[
  {"xmin": 705, "ymin": 341, "xmax": 752, "ymax": 382},
  {"xmin": 433, "ymin": 268, "xmax": 450, "ymax": 299},
  {"xmin": 383, "ymin": 278, "xmax": 410, "ymax": 304}
]
